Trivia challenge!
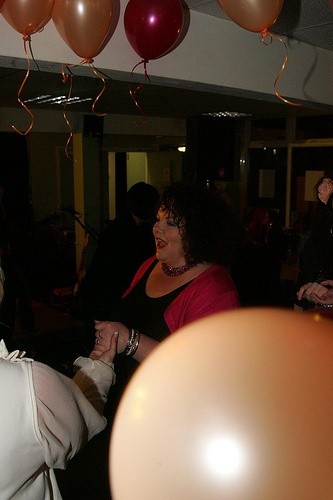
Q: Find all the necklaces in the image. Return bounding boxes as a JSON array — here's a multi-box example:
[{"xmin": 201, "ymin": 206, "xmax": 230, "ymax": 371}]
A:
[{"xmin": 161, "ymin": 259, "xmax": 199, "ymax": 276}]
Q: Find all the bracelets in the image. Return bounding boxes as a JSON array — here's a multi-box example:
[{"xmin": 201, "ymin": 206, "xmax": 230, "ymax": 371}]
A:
[{"xmin": 119, "ymin": 328, "xmax": 143, "ymax": 358}]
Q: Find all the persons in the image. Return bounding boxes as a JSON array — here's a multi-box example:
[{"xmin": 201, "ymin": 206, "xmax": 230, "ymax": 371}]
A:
[
  {"xmin": 1, "ymin": 186, "xmax": 42, "ymax": 342},
  {"xmin": 292, "ymin": 174, "xmax": 333, "ymax": 310},
  {"xmin": 92, "ymin": 180, "xmax": 243, "ymax": 500},
  {"xmin": 295, "ymin": 279, "xmax": 333, "ymax": 309},
  {"xmin": 0, "ymin": 267, "xmax": 121, "ymax": 500},
  {"xmin": 77, "ymin": 182, "xmax": 162, "ymax": 359}
]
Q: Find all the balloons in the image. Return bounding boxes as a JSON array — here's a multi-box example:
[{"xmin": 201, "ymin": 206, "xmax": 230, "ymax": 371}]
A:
[
  {"xmin": 0, "ymin": 0, "xmax": 54, "ymax": 42},
  {"xmin": 107, "ymin": 307, "xmax": 333, "ymax": 500},
  {"xmin": 123, "ymin": 0, "xmax": 185, "ymax": 63},
  {"xmin": 50, "ymin": 0, "xmax": 115, "ymax": 64},
  {"xmin": 217, "ymin": 0, "xmax": 284, "ymax": 36}
]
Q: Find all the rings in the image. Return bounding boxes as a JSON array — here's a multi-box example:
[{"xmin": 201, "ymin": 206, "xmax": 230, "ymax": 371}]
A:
[
  {"xmin": 98, "ymin": 331, "xmax": 103, "ymax": 338},
  {"xmin": 97, "ymin": 339, "xmax": 99, "ymax": 344}
]
